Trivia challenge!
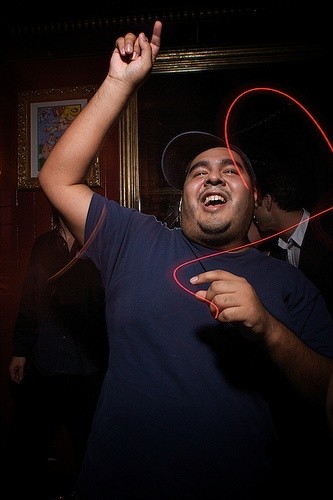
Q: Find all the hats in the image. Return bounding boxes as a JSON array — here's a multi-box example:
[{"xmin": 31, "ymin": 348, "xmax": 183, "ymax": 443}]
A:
[{"xmin": 160, "ymin": 131, "xmax": 257, "ymax": 191}]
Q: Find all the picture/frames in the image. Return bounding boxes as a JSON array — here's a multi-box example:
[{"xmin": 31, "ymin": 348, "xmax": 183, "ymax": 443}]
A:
[{"xmin": 15, "ymin": 85, "xmax": 105, "ymax": 191}]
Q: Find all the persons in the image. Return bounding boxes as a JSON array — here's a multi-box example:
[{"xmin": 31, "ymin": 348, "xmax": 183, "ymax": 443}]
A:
[
  {"xmin": 9, "ymin": 206, "xmax": 109, "ymax": 438},
  {"xmin": 38, "ymin": 21, "xmax": 333, "ymax": 500},
  {"xmin": 254, "ymin": 154, "xmax": 333, "ymax": 317}
]
